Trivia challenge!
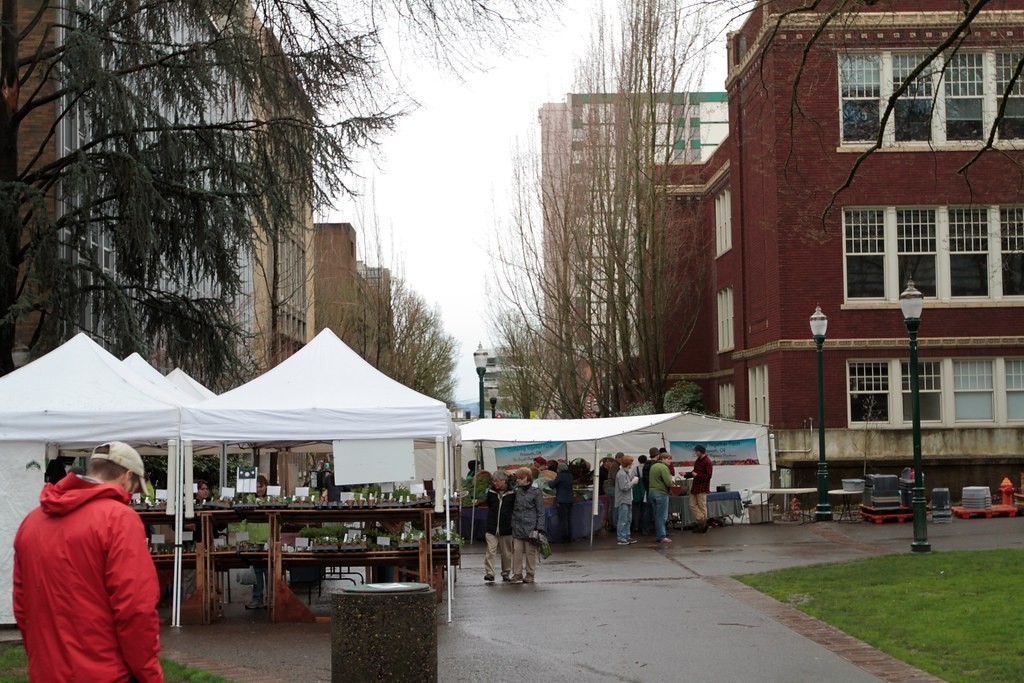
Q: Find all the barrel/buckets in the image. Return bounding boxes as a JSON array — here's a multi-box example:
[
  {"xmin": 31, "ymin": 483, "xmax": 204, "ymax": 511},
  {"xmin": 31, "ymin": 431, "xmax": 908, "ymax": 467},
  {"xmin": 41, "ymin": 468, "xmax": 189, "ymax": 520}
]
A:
[{"xmin": 674, "ymin": 477, "xmax": 694, "ymax": 495}]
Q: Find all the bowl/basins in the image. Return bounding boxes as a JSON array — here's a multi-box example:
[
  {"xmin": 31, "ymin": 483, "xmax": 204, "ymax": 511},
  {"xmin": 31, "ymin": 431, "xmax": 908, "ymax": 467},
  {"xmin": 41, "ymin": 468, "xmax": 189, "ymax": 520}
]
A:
[{"xmin": 670, "ymin": 486, "xmax": 683, "ymax": 496}]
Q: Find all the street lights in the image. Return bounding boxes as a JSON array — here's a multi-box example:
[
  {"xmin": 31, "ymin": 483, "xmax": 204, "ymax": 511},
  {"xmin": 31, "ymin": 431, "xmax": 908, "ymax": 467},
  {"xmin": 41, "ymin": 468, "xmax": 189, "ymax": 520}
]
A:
[
  {"xmin": 809, "ymin": 303, "xmax": 833, "ymax": 519},
  {"xmin": 473, "ymin": 341, "xmax": 488, "ymax": 418},
  {"xmin": 487, "ymin": 379, "xmax": 499, "ymax": 418},
  {"xmin": 899, "ymin": 277, "xmax": 932, "ymax": 550}
]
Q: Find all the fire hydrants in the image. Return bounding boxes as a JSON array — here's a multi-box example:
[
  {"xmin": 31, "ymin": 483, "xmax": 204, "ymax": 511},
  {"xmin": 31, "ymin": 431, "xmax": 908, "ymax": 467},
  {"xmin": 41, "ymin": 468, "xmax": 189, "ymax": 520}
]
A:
[{"xmin": 999, "ymin": 478, "xmax": 1014, "ymax": 504}]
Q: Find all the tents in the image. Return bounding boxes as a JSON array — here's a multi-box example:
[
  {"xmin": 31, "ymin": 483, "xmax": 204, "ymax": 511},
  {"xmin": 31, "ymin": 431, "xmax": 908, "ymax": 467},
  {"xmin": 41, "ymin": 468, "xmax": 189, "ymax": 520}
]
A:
[
  {"xmin": 460, "ymin": 411, "xmax": 775, "ymax": 551},
  {"xmin": 0, "ymin": 326, "xmax": 460, "ymax": 628}
]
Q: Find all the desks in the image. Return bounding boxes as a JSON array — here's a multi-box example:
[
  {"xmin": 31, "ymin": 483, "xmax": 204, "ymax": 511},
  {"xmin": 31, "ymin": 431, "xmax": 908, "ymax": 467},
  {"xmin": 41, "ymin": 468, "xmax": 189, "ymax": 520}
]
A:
[
  {"xmin": 544, "ymin": 499, "xmax": 605, "ymax": 544},
  {"xmin": 753, "ymin": 488, "xmax": 817, "ymax": 525},
  {"xmin": 830, "ymin": 489, "xmax": 861, "ymax": 522},
  {"xmin": 665, "ymin": 491, "xmax": 743, "ymax": 534}
]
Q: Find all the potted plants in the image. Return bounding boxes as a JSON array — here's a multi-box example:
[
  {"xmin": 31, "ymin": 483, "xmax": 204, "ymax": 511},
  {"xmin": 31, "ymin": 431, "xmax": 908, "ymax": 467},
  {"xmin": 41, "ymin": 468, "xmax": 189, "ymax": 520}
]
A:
[{"xmin": 211, "ymin": 481, "xmax": 464, "ymax": 551}]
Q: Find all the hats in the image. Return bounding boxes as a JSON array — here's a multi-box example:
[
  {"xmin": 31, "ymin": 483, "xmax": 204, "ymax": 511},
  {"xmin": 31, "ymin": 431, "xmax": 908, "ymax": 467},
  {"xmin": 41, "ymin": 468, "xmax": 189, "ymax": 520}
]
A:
[
  {"xmin": 90, "ymin": 441, "xmax": 150, "ymax": 498},
  {"xmin": 649, "ymin": 447, "xmax": 660, "ymax": 457}
]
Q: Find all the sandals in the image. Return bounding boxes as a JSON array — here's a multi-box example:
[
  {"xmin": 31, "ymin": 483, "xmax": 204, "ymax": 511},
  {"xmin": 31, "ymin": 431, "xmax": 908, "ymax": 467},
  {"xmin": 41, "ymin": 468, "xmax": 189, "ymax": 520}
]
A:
[{"xmin": 692, "ymin": 528, "xmax": 700, "ymax": 532}]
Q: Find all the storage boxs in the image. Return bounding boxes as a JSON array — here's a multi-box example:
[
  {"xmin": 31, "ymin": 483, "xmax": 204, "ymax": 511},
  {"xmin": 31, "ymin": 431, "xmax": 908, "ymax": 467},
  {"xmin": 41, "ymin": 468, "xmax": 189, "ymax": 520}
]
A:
[
  {"xmin": 842, "ymin": 475, "xmax": 992, "ymax": 525},
  {"xmin": 749, "ymin": 503, "xmax": 772, "ymax": 524}
]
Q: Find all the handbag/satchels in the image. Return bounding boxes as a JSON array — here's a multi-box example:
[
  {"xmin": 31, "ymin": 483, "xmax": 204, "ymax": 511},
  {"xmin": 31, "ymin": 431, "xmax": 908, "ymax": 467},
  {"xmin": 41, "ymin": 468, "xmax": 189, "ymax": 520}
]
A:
[{"xmin": 235, "ymin": 565, "xmax": 258, "ymax": 584}]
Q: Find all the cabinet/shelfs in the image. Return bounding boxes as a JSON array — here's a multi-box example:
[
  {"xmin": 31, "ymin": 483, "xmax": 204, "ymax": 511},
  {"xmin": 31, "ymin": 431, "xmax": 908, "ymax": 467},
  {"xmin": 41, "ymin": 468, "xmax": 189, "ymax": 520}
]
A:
[{"xmin": 130, "ymin": 498, "xmax": 457, "ymax": 624}]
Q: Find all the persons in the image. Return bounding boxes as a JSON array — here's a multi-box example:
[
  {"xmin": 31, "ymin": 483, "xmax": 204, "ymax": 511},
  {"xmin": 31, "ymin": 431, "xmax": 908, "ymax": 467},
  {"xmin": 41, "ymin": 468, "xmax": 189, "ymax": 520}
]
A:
[
  {"xmin": 324, "ymin": 469, "xmax": 342, "ymax": 501},
  {"xmin": 482, "ymin": 467, "xmax": 545, "ymax": 583},
  {"xmin": 466, "ymin": 460, "xmax": 481, "ymax": 477},
  {"xmin": 679, "ymin": 445, "xmax": 712, "ymax": 534},
  {"xmin": 530, "ymin": 457, "xmax": 574, "ymax": 544},
  {"xmin": 240, "ymin": 475, "xmax": 274, "ymax": 609},
  {"xmin": 12, "ymin": 442, "xmax": 164, "ymax": 683},
  {"xmin": 192, "ymin": 479, "xmax": 219, "ymax": 542},
  {"xmin": 598, "ymin": 447, "xmax": 675, "ymax": 545}
]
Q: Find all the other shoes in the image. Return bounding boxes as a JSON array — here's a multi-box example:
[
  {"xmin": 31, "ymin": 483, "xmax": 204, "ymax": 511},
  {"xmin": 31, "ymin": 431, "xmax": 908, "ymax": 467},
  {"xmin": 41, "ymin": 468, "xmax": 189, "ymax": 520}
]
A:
[
  {"xmin": 617, "ymin": 539, "xmax": 629, "ymax": 545},
  {"xmin": 702, "ymin": 522, "xmax": 707, "ymax": 533},
  {"xmin": 658, "ymin": 537, "xmax": 672, "ymax": 543},
  {"xmin": 502, "ymin": 575, "xmax": 510, "ymax": 582},
  {"xmin": 524, "ymin": 573, "xmax": 535, "ymax": 583},
  {"xmin": 246, "ymin": 601, "xmax": 263, "ymax": 609},
  {"xmin": 507, "ymin": 574, "xmax": 524, "ymax": 583},
  {"xmin": 484, "ymin": 574, "xmax": 494, "ymax": 581},
  {"xmin": 627, "ymin": 538, "xmax": 638, "ymax": 544}
]
